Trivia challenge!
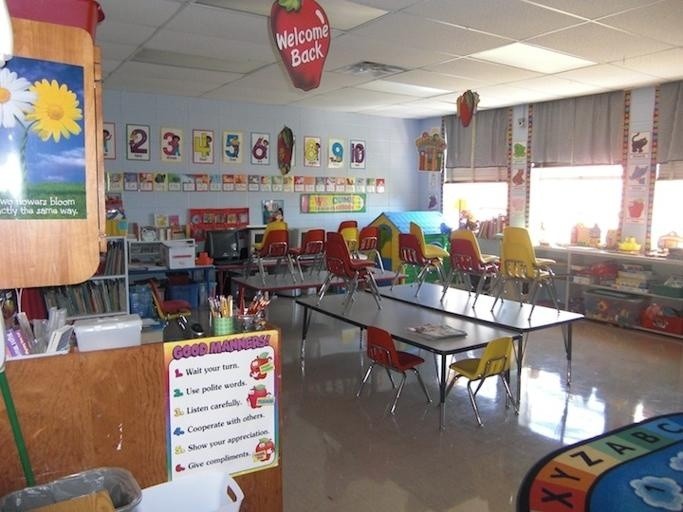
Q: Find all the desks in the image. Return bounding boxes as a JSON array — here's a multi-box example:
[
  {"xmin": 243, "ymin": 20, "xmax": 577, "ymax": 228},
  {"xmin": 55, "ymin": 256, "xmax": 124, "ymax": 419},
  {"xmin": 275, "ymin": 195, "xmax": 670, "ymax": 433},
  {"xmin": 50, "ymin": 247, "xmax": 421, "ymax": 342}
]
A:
[
  {"xmin": 213, "ymin": 261, "xmax": 301, "ymax": 300},
  {"xmin": 296, "ymin": 293, "xmax": 523, "ymax": 431},
  {"xmin": 231, "ymin": 267, "xmax": 409, "ymax": 316},
  {"xmin": 364, "ymin": 282, "xmax": 585, "ymax": 405}
]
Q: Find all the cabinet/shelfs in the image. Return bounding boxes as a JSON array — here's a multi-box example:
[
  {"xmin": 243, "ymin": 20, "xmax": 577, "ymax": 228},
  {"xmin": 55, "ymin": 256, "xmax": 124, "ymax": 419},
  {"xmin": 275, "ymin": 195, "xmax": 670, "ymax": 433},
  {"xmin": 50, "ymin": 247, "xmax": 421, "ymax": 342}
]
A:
[
  {"xmin": 535, "ymin": 244, "xmax": 568, "ymax": 310},
  {"xmin": 45, "ymin": 235, "xmax": 129, "ymax": 322},
  {"xmin": 128, "ymin": 239, "xmax": 215, "ymax": 323},
  {"xmin": 564, "ymin": 243, "xmax": 683, "ymax": 338},
  {"xmin": 0, "ymin": 17, "xmax": 106, "ymax": 289}
]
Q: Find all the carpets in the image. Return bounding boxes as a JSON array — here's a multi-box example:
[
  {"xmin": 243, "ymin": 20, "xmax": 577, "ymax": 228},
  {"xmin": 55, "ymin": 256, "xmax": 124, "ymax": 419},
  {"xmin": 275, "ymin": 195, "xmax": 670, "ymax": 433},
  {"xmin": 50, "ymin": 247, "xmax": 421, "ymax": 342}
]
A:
[{"xmin": 516, "ymin": 412, "xmax": 683, "ymax": 512}]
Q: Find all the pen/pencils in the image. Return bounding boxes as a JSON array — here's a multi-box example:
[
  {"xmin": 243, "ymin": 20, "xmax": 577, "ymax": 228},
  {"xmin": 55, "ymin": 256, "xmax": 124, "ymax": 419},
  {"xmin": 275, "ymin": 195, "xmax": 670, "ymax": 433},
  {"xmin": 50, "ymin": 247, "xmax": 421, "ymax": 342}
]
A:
[
  {"xmin": 242, "ymin": 291, "xmax": 272, "ymax": 315},
  {"xmin": 208, "ymin": 295, "xmax": 233, "ymax": 318}
]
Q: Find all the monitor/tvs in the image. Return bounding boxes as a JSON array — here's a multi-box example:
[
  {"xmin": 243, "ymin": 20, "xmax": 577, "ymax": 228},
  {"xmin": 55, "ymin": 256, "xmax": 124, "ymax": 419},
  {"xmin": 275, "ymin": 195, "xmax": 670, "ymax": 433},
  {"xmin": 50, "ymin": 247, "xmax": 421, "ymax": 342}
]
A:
[
  {"xmin": 297, "ymin": 229, "xmax": 308, "ymax": 249},
  {"xmin": 204, "ymin": 230, "xmax": 240, "ymax": 258},
  {"xmin": 248, "ymin": 229, "xmax": 265, "ymax": 252}
]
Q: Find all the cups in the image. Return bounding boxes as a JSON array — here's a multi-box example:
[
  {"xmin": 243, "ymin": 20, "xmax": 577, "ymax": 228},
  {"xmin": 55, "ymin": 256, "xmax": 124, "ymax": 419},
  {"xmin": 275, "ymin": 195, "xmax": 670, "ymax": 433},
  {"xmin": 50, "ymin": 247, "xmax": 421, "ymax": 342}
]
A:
[{"xmin": 235, "ymin": 308, "xmax": 262, "ymax": 333}]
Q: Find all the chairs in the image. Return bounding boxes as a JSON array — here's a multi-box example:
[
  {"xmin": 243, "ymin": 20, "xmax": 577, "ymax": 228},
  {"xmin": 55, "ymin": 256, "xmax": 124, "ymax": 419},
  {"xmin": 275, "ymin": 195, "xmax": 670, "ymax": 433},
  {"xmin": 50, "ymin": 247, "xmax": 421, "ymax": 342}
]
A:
[
  {"xmin": 357, "ymin": 326, "xmax": 433, "ymax": 413},
  {"xmin": 439, "ymin": 337, "xmax": 519, "ymax": 427},
  {"xmin": 150, "ymin": 279, "xmax": 193, "ymax": 325},
  {"xmin": 151, "ymin": 291, "xmax": 192, "ymax": 331}
]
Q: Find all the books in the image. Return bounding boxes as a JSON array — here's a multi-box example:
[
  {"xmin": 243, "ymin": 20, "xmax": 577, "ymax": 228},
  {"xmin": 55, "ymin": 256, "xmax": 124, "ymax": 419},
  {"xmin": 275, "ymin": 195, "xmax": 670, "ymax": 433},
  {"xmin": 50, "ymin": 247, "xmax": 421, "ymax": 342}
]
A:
[
  {"xmin": 43, "ymin": 242, "xmax": 126, "ymax": 317},
  {"xmin": 477, "ymin": 214, "xmax": 502, "ymax": 239}
]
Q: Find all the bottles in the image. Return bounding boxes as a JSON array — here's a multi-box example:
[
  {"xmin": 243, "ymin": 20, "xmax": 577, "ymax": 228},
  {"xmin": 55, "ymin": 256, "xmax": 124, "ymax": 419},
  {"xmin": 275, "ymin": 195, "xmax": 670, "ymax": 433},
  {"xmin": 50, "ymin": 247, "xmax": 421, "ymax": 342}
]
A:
[{"xmin": 120, "ymin": 222, "xmax": 128, "ymax": 238}]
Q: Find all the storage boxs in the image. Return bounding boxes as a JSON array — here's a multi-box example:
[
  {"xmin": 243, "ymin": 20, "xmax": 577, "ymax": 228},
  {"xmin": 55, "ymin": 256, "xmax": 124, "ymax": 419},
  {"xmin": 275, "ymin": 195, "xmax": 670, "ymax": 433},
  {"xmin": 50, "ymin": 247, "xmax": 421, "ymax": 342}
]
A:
[
  {"xmin": 583, "ymin": 289, "xmax": 644, "ymax": 325},
  {"xmin": 73, "ymin": 313, "xmax": 142, "ymax": 353},
  {"xmin": 6, "ymin": 0, "xmax": 105, "ymax": 44},
  {"xmin": 162, "ymin": 280, "xmax": 198, "ymax": 310},
  {"xmin": 639, "ymin": 308, "xmax": 683, "ymax": 336}
]
